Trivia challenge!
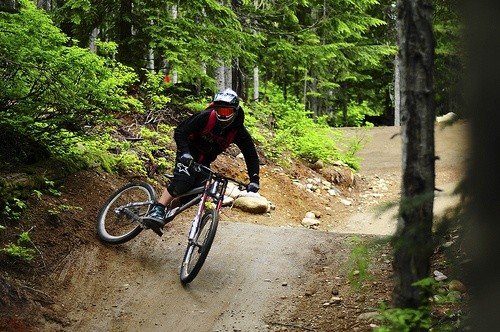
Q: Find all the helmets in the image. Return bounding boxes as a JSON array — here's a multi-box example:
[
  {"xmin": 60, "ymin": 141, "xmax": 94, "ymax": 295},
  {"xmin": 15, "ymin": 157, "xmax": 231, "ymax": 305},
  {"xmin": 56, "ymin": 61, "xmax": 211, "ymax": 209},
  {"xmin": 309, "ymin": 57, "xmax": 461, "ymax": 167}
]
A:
[{"xmin": 214, "ymin": 88, "xmax": 239, "ymax": 128}]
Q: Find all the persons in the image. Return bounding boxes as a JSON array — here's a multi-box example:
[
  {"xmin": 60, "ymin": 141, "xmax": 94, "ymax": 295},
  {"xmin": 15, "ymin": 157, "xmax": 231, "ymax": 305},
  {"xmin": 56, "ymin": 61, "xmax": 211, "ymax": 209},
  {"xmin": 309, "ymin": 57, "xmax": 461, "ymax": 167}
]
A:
[{"xmin": 143, "ymin": 88, "xmax": 260, "ymax": 235}]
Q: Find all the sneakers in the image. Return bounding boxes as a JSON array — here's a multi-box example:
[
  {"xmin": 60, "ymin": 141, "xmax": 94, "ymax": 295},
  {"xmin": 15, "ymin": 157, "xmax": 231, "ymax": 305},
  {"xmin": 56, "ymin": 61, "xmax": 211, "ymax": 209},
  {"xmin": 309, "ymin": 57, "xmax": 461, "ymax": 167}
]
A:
[{"xmin": 141, "ymin": 205, "xmax": 164, "ymax": 228}]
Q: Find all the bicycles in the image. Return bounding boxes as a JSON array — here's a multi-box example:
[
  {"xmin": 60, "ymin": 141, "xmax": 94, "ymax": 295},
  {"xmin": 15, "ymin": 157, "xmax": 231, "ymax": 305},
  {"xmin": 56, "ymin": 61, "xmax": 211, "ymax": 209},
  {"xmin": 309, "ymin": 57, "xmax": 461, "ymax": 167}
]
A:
[{"xmin": 95, "ymin": 160, "xmax": 250, "ymax": 283}]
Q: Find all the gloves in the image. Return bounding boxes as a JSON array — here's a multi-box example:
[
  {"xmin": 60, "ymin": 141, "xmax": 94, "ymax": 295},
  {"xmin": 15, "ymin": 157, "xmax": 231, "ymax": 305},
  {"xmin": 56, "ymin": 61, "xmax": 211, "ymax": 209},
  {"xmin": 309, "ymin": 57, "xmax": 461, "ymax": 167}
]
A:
[
  {"xmin": 246, "ymin": 175, "xmax": 260, "ymax": 193},
  {"xmin": 181, "ymin": 150, "xmax": 194, "ymax": 168}
]
